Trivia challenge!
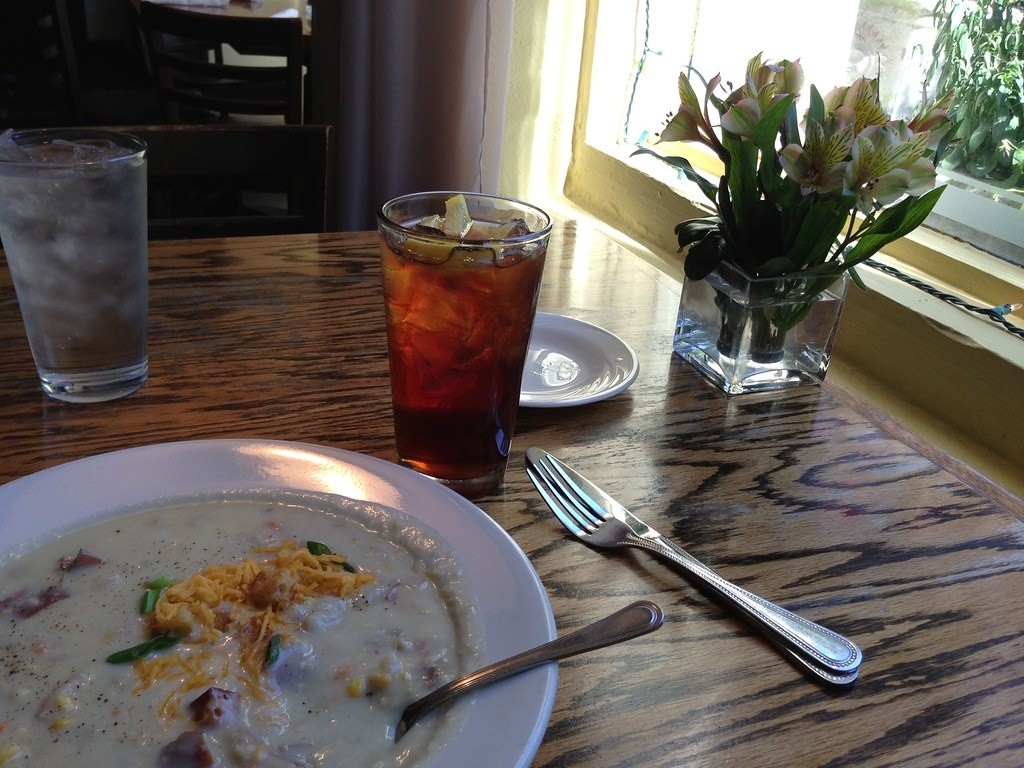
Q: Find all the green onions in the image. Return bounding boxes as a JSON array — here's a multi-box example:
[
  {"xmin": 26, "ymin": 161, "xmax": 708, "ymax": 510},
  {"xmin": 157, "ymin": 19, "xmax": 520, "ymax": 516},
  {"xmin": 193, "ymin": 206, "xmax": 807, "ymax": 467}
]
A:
[{"xmin": 105, "ymin": 542, "xmax": 356, "ymax": 669}]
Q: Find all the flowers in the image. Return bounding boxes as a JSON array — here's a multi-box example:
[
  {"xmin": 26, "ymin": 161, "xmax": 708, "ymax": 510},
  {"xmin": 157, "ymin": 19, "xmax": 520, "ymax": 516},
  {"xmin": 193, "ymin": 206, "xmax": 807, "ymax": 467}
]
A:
[{"xmin": 632, "ymin": 42, "xmax": 968, "ymax": 360}]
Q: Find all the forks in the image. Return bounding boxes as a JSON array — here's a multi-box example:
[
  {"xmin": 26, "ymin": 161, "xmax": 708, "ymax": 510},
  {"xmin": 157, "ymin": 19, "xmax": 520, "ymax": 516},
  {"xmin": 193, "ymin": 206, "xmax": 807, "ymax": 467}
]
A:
[{"xmin": 527, "ymin": 455, "xmax": 862, "ymax": 674}]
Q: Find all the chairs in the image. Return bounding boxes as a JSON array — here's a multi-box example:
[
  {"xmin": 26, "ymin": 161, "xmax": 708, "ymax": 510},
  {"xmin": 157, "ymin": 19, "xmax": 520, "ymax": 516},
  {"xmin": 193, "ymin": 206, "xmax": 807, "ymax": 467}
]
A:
[
  {"xmin": 0, "ymin": 122, "xmax": 336, "ymax": 249},
  {"xmin": 139, "ymin": 1, "xmax": 301, "ymax": 127}
]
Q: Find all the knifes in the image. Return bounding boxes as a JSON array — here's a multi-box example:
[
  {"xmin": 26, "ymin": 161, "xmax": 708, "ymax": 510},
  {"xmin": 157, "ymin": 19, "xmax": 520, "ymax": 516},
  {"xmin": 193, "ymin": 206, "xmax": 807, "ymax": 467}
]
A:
[{"xmin": 526, "ymin": 445, "xmax": 858, "ymax": 688}]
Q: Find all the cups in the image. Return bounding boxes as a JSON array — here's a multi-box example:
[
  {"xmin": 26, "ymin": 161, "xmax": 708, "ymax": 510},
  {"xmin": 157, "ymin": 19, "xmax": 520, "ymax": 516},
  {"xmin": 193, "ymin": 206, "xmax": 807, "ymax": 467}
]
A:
[
  {"xmin": 374, "ymin": 192, "xmax": 554, "ymax": 497},
  {"xmin": 0, "ymin": 128, "xmax": 150, "ymax": 404}
]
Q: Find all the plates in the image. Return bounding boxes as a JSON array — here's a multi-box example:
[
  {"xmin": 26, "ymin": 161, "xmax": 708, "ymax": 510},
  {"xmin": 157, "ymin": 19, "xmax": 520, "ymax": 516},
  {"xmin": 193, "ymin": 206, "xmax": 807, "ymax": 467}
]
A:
[
  {"xmin": 1, "ymin": 438, "xmax": 558, "ymax": 768},
  {"xmin": 518, "ymin": 311, "xmax": 641, "ymax": 407}
]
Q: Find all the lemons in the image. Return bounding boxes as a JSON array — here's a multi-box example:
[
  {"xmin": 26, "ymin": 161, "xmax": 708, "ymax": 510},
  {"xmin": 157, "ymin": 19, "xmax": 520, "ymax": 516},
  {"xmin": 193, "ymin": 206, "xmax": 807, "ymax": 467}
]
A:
[{"xmin": 404, "ymin": 194, "xmax": 516, "ymax": 263}]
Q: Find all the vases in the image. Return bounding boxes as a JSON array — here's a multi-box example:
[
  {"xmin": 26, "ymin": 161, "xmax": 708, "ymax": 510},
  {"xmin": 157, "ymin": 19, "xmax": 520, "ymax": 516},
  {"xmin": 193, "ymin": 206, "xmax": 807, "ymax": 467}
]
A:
[{"xmin": 676, "ymin": 234, "xmax": 852, "ymax": 395}]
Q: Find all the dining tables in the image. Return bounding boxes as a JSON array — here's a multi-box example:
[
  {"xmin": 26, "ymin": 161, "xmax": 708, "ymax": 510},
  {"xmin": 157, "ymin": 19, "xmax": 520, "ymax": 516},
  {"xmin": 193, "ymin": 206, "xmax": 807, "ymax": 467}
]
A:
[{"xmin": 0, "ymin": 203, "xmax": 1024, "ymax": 768}]
